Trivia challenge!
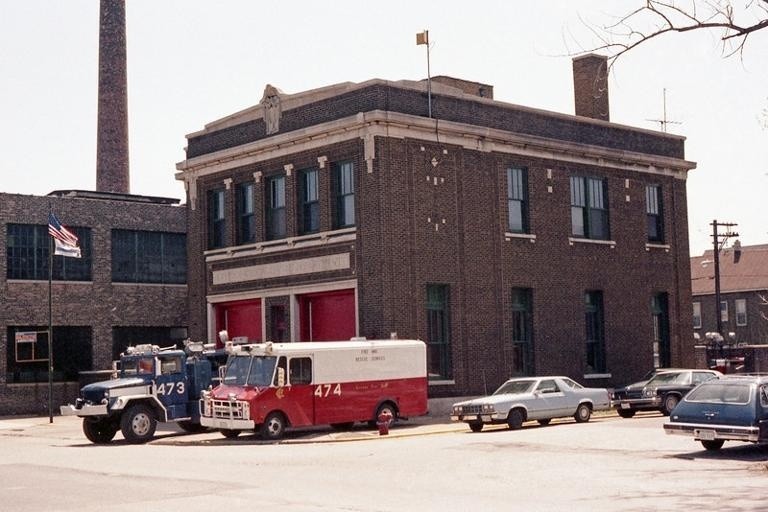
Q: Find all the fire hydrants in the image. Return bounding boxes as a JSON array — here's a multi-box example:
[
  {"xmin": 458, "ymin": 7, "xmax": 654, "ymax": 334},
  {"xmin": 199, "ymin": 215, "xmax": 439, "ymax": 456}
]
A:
[{"xmin": 376, "ymin": 413, "xmax": 391, "ymax": 435}]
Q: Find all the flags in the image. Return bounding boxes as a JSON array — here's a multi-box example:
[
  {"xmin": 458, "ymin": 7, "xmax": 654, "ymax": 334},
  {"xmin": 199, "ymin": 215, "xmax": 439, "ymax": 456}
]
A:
[
  {"xmin": 52, "ymin": 237, "xmax": 83, "ymax": 259},
  {"xmin": 48, "ymin": 213, "xmax": 78, "ymax": 245}
]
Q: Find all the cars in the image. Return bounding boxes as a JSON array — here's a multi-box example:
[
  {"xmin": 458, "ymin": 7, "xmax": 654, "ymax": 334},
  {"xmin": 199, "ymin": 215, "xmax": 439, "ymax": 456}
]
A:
[
  {"xmin": 663, "ymin": 373, "xmax": 767, "ymax": 453},
  {"xmin": 451, "ymin": 376, "xmax": 610, "ymax": 431},
  {"xmin": 608, "ymin": 365, "xmax": 723, "ymax": 420}
]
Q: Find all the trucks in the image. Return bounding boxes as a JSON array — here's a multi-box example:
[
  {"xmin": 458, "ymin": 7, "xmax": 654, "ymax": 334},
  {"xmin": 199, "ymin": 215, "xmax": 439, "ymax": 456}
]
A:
[
  {"xmin": 72, "ymin": 338, "xmax": 233, "ymax": 445},
  {"xmin": 198, "ymin": 337, "xmax": 429, "ymax": 440}
]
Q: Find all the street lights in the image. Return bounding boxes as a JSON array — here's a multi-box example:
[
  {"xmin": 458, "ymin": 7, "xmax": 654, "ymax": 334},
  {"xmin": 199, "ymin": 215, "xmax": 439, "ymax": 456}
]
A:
[{"xmin": 692, "ymin": 329, "xmax": 738, "ymax": 376}]
